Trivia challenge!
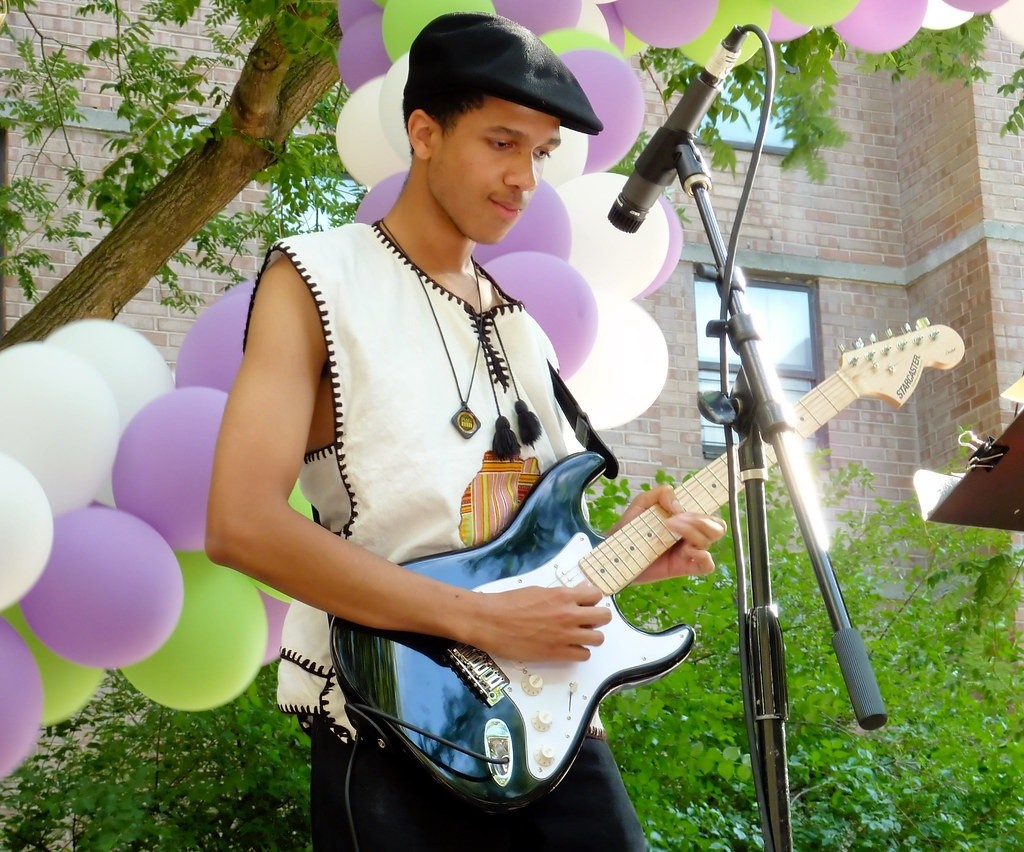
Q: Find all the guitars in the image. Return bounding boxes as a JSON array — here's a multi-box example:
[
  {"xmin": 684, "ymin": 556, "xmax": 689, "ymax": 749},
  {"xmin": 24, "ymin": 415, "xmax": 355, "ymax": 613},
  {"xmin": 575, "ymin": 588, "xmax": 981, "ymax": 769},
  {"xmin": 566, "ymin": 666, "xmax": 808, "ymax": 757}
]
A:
[{"xmin": 328, "ymin": 312, "xmax": 972, "ymax": 816}]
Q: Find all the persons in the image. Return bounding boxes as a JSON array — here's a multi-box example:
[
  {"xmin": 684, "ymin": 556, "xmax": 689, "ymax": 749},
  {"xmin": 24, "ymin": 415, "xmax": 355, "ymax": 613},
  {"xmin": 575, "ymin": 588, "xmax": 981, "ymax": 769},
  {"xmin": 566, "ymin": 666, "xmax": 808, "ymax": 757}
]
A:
[{"xmin": 204, "ymin": 14, "xmax": 731, "ymax": 852}]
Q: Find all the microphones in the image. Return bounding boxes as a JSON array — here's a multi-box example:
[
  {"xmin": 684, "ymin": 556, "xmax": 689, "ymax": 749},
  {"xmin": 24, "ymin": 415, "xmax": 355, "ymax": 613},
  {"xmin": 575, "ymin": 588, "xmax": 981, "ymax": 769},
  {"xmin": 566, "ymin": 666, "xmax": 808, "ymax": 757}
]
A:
[{"xmin": 608, "ymin": 26, "xmax": 748, "ymax": 233}]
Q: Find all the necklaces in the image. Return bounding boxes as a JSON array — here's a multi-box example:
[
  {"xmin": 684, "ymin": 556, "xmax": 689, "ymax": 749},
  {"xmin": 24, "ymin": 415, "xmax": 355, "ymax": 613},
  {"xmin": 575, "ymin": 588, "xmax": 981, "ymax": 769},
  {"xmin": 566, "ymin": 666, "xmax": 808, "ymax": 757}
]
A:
[{"xmin": 380, "ymin": 216, "xmax": 481, "ymax": 439}]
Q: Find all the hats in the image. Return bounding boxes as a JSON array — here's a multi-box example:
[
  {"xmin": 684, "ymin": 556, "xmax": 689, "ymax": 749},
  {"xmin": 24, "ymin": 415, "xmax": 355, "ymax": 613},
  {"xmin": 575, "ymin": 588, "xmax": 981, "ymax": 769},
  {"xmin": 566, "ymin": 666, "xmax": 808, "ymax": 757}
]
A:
[{"xmin": 408, "ymin": 12, "xmax": 603, "ymax": 137}]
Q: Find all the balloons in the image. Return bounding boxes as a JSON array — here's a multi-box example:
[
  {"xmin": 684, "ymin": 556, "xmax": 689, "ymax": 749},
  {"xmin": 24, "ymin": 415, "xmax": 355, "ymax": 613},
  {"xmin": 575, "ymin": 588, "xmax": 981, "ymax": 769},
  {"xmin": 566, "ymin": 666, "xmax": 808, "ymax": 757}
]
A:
[
  {"xmin": 332, "ymin": 0, "xmax": 1024, "ymax": 434},
  {"xmin": 0, "ymin": 275, "xmax": 312, "ymax": 773}
]
